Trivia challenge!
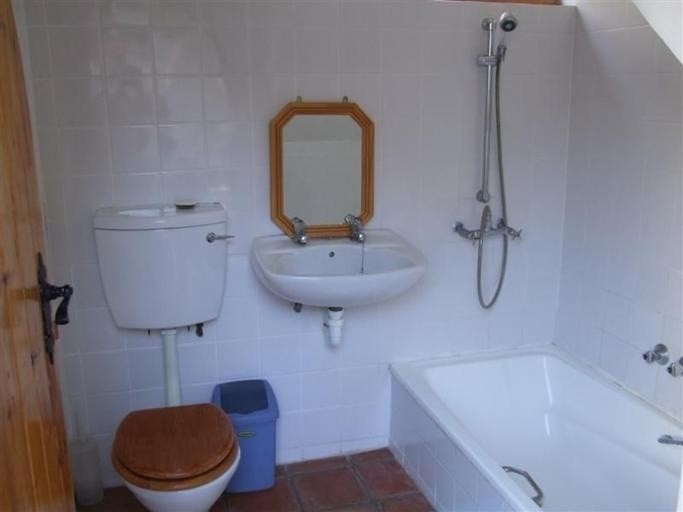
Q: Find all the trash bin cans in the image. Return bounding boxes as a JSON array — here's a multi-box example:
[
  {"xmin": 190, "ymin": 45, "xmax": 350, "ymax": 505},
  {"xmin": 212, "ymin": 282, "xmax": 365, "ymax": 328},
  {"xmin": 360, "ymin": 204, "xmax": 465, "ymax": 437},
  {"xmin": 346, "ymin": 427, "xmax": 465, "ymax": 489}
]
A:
[{"xmin": 211, "ymin": 379, "xmax": 279, "ymax": 494}]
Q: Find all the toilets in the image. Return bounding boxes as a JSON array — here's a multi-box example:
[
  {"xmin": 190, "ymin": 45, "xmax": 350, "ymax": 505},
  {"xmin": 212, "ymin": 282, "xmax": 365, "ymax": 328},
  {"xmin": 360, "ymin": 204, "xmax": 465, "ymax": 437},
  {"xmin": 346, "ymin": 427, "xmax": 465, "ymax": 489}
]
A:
[{"xmin": 92, "ymin": 204, "xmax": 241, "ymax": 512}]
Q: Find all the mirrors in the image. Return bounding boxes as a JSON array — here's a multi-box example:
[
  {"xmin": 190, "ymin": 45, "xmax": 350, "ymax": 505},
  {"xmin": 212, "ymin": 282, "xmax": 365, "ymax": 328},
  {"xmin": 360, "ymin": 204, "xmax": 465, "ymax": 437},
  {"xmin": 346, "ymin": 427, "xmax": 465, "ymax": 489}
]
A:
[{"xmin": 271, "ymin": 95, "xmax": 376, "ymax": 241}]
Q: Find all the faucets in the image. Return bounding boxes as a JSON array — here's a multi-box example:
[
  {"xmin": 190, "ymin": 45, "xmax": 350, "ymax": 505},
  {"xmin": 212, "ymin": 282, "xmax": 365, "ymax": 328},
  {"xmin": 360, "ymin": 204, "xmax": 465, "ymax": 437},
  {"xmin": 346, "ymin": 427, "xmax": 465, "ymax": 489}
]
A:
[
  {"xmin": 291, "ymin": 217, "xmax": 310, "ymax": 246},
  {"xmin": 350, "ymin": 217, "xmax": 367, "ymax": 242},
  {"xmin": 656, "ymin": 433, "xmax": 683, "ymax": 447}
]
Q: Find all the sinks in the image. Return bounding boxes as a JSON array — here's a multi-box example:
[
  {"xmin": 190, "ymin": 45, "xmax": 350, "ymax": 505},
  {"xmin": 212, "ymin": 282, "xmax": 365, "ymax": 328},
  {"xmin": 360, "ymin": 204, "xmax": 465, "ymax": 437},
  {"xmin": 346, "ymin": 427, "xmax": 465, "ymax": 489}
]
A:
[{"xmin": 251, "ymin": 230, "xmax": 427, "ymax": 309}]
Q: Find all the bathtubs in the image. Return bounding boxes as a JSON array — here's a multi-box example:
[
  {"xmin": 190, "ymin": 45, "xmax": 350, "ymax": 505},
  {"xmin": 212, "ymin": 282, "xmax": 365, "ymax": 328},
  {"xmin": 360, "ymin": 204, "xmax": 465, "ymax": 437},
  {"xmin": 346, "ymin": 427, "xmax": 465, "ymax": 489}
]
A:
[{"xmin": 389, "ymin": 344, "xmax": 683, "ymax": 511}]
